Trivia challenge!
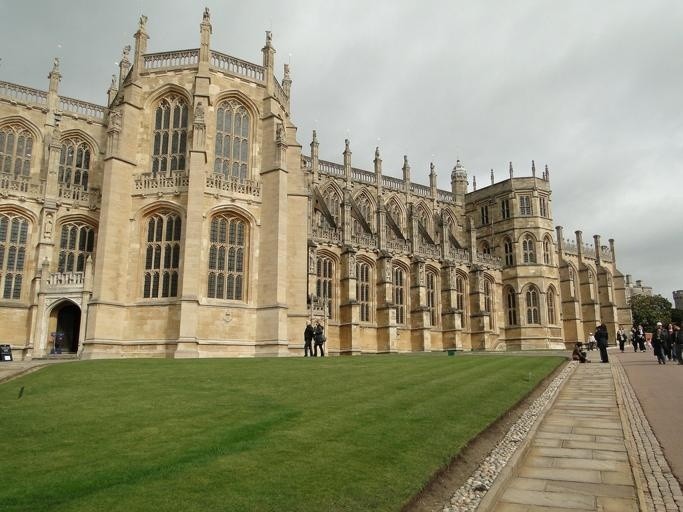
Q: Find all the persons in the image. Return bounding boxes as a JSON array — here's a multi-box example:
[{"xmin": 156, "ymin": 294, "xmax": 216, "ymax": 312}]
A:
[
  {"xmin": 616, "ymin": 325, "xmax": 627, "ymax": 351},
  {"xmin": 586, "ymin": 331, "xmax": 596, "ymax": 351},
  {"xmin": 628, "ymin": 320, "xmax": 683, "ymax": 365},
  {"xmin": 302, "ymin": 320, "xmax": 315, "ymax": 357},
  {"xmin": 571, "ymin": 342, "xmax": 592, "ymax": 363},
  {"xmin": 594, "ymin": 323, "xmax": 609, "ymax": 363},
  {"xmin": 311, "ymin": 319, "xmax": 326, "ymax": 356}
]
